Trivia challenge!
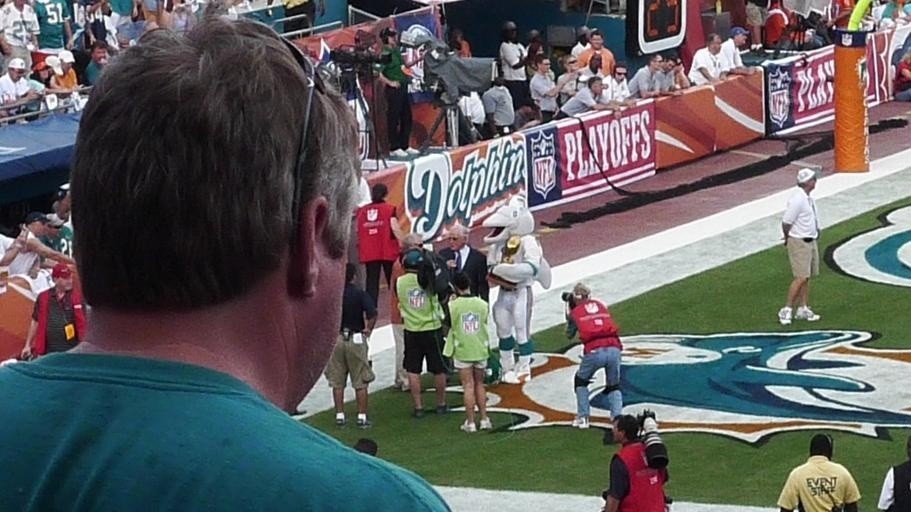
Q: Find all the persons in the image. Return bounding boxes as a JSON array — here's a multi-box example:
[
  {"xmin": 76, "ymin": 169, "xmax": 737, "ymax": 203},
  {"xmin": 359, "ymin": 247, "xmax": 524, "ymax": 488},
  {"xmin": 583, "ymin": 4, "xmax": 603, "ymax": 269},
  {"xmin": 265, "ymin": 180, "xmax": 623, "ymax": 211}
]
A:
[
  {"xmin": 776, "ymin": 433, "xmax": 862, "ymax": 512},
  {"xmin": 356, "ymin": 184, "xmax": 405, "ymax": 317},
  {"xmin": 324, "ymin": 264, "xmax": 379, "ymax": 429},
  {"xmin": 777, "ymin": 167, "xmax": 821, "ymax": 326},
  {"xmin": 284, "ymin": 1, "xmax": 316, "ymax": 38},
  {"xmin": 565, "ymin": 282, "xmax": 623, "ymax": 430},
  {"xmin": 376, "ymin": 21, "xmax": 420, "ymax": 160},
  {"xmin": 0, "ymin": 2, "xmax": 233, "ymax": 128},
  {"xmin": 0, "ymin": 1, "xmax": 450, "ymax": 511},
  {"xmin": 348, "ymin": 173, "xmax": 373, "ymax": 288},
  {"xmin": 877, "ymin": 436, "xmax": 911, "ymax": 512},
  {"xmin": 447, "ymin": 1, "xmax": 692, "ymax": 147},
  {"xmin": 602, "ymin": 414, "xmax": 672, "ymax": 512},
  {"xmin": 893, "ymin": 53, "xmax": 910, "ymax": 103},
  {"xmin": 389, "ymin": 224, "xmax": 493, "ymax": 434},
  {"xmin": 0, "ymin": 183, "xmax": 90, "ymax": 362},
  {"xmin": 689, "ymin": 28, "xmax": 758, "ymax": 85},
  {"xmin": 745, "ymin": 1, "xmax": 910, "ymax": 54}
]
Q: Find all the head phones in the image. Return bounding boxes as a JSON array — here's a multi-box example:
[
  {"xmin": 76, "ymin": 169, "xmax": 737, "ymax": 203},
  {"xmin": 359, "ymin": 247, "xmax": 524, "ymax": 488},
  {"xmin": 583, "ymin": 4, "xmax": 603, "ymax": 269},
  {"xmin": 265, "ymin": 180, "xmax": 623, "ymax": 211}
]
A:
[{"xmin": 382, "ymin": 27, "xmax": 389, "ymax": 44}]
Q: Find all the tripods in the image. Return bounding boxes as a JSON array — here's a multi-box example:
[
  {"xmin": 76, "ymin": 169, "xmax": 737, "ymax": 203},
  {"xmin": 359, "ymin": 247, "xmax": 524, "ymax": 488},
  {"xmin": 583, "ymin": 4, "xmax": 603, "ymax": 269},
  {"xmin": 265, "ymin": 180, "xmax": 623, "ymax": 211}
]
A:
[{"xmin": 339, "ymin": 71, "xmax": 388, "ymax": 171}]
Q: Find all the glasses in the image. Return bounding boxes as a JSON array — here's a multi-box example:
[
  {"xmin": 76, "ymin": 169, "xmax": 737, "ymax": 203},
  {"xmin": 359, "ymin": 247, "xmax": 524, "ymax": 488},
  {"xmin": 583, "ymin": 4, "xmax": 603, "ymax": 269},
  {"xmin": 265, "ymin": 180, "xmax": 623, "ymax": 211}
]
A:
[
  {"xmin": 616, "ymin": 72, "xmax": 627, "ymax": 75},
  {"xmin": 281, "ymin": 34, "xmax": 326, "ymax": 256},
  {"xmin": 568, "ymin": 60, "xmax": 577, "ymax": 63}
]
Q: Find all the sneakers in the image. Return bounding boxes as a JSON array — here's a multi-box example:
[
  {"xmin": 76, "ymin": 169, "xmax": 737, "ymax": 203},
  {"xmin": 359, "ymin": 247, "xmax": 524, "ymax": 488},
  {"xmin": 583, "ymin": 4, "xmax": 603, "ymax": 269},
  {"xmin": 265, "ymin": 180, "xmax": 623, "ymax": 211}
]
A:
[
  {"xmin": 356, "ymin": 419, "xmax": 372, "ymax": 429},
  {"xmin": 777, "ymin": 306, "xmax": 792, "ymax": 325},
  {"xmin": 335, "ymin": 418, "xmax": 350, "ymax": 427},
  {"xmin": 405, "ymin": 147, "xmax": 420, "ymax": 154},
  {"xmin": 394, "ymin": 379, "xmax": 491, "ymax": 432},
  {"xmin": 571, "ymin": 416, "xmax": 591, "ymax": 429},
  {"xmin": 389, "ymin": 148, "xmax": 409, "ymax": 157},
  {"xmin": 794, "ymin": 306, "xmax": 821, "ymax": 321}
]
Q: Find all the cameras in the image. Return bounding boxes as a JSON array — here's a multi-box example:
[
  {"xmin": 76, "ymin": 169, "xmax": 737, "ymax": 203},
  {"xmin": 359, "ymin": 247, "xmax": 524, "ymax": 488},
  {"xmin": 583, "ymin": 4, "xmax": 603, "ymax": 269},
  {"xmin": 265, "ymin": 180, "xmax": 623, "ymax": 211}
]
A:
[
  {"xmin": 562, "ymin": 291, "xmax": 576, "ymax": 309},
  {"xmin": 636, "ymin": 410, "xmax": 668, "ymax": 468}
]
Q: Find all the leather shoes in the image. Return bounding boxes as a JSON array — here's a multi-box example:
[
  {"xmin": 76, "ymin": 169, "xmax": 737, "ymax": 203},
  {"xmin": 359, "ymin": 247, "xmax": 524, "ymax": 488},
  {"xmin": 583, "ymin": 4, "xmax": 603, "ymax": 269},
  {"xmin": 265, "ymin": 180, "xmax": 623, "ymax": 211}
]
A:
[{"xmin": 291, "ymin": 408, "xmax": 306, "ymax": 415}]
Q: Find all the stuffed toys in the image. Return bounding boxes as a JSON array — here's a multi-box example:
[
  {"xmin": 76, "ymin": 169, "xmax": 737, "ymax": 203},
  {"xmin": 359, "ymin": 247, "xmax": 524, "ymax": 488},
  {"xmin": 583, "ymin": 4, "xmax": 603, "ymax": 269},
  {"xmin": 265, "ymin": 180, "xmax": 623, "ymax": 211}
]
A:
[{"xmin": 482, "ymin": 197, "xmax": 551, "ymax": 385}]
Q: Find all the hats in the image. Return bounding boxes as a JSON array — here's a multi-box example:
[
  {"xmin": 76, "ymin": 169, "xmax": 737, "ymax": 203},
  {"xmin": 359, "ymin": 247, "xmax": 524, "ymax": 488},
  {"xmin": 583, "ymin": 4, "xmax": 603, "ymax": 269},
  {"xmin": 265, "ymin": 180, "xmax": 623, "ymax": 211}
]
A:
[
  {"xmin": 25, "ymin": 211, "xmax": 51, "ymax": 225},
  {"xmin": 730, "ymin": 27, "xmax": 749, "ymax": 38},
  {"xmin": 797, "ymin": 168, "xmax": 816, "ymax": 185},
  {"xmin": 406, "ymin": 250, "xmax": 423, "ymax": 266},
  {"xmin": 378, "ymin": 27, "xmax": 396, "ymax": 37},
  {"xmin": 7, "ymin": 50, "xmax": 75, "ymax": 75},
  {"xmin": 576, "ymin": 25, "xmax": 597, "ymax": 36},
  {"xmin": 51, "ymin": 261, "xmax": 71, "ymax": 277},
  {"xmin": 46, "ymin": 213, "xmax": 64, "ymax": 227}
]
